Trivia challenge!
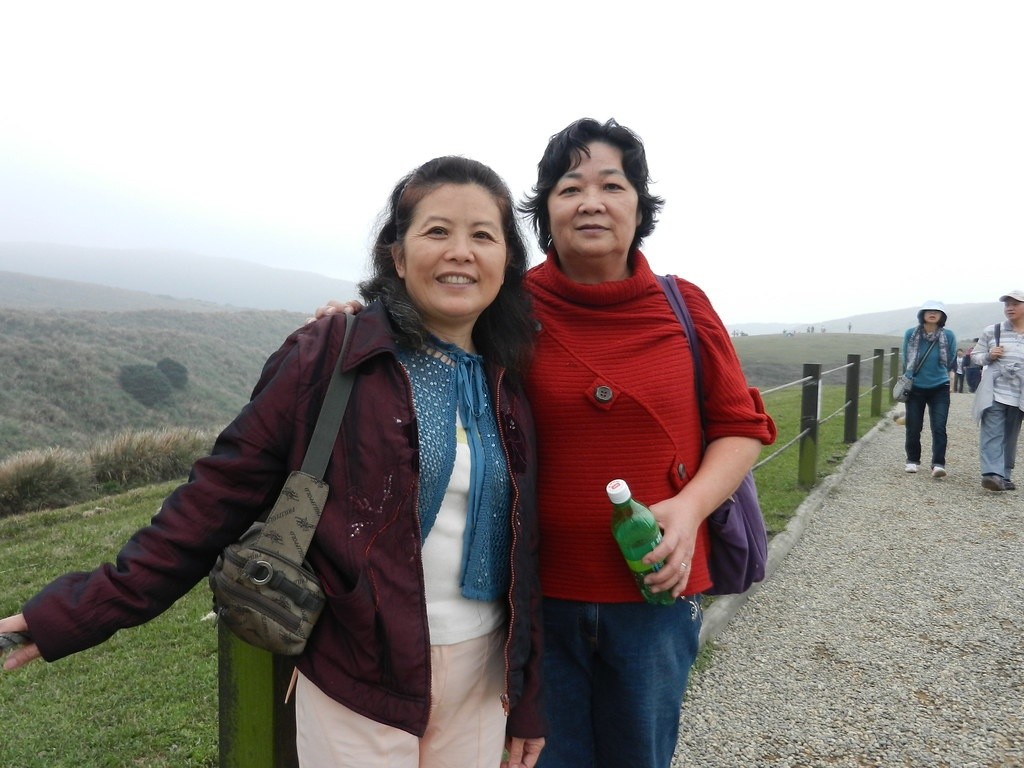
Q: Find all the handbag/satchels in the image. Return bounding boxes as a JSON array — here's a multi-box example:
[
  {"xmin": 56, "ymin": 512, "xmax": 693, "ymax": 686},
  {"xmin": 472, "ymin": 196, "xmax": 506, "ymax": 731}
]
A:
[
  {"xmin": 655, "ymin": 269, "xmax": 768, "ymax": 595},
  {"xmin": 893, "ymin": 375, "xmax": 912, "ymax": 402},
  {"xmin": 207, "ymin": 311, "xmax": 359, "ymax": 656}
]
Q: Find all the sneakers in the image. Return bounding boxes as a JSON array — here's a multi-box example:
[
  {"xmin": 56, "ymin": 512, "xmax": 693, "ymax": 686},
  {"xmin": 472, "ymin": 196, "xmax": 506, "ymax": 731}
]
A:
[
  {"xmin": 932, "ymin": 466, "xmax": 947, "ymax": 477},
  {"xmin": 1004, "ymin": 479, "xmax": 1015, "ymax": 490},
  {"xmin": 905, "ymin": 463, "xmax": 917, "ymax": 473},
  {"xmin": 981, "ymin": 474, "xmax": 1005, "ymax": 491}
]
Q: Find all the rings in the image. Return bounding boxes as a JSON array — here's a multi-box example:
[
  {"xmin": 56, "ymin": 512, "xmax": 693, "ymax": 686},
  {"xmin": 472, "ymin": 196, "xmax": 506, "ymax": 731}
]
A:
[{"xmin": 681, "ymin": 562, "xmax": 687, "ymax": 567}]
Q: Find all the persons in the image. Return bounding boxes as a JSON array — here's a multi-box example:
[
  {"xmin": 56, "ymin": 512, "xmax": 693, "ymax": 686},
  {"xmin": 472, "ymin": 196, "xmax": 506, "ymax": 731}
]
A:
[
  {"xmin": 970, "ymin": 290, "xmax": 1024, "ymax": 490},
  {"xmin": 807, "ymin": 325, "xmax": 825, "ymax": 335},
  {"xmin": 0, "ymin": 157, "xmax": 549, "ymax": 768},
  {"xmin": 903, "ymin": 301, "xmax": 956, "ymax": 477},
  {"xmin": 305, "ymin": 120, "xmax": 778, "ymax": 768},
  {"xmin": 954, "ymin": 348, "xmax": 983, "ymax": 393}
]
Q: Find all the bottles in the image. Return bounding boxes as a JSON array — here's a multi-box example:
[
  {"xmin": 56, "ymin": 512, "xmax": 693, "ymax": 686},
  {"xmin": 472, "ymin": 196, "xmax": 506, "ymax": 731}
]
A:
[
  {"xmin": 904, "ymin": 364, "xmax": 913, "ymax": 379},
  {"xmin": 607, "ymin": 479, "xmax": 677, "ymax": 606}
]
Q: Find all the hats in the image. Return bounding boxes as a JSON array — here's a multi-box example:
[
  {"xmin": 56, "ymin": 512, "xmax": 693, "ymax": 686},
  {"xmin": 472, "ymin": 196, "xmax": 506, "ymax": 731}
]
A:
[
  {"xmin": 999, "ymin": 290, "xmax": 1024, "ymax": 303},
  {"xmin": 917, "ymin": 301, "xmax": 947, "ymax": 325}
]
